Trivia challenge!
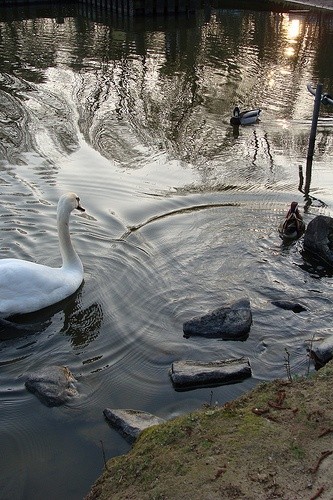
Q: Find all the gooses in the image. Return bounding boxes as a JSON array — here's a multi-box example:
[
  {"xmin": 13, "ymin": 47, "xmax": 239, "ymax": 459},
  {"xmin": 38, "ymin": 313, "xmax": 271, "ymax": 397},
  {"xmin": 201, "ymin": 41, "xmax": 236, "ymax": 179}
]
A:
[
  {"xmin": 280, "ymin": 202, "xmax": 305, "ymax": 240},
  {"xmin": 0, "ymin": 193, "xmax": 85, "ymax": 314},
  {"xmin": 233, "ymin": 106, "xmax": 262, "ymax": 125}
]
